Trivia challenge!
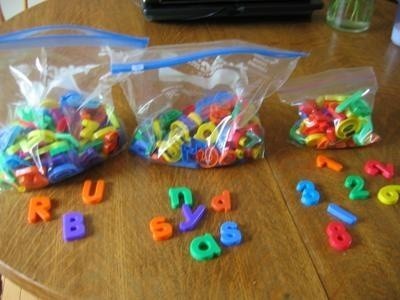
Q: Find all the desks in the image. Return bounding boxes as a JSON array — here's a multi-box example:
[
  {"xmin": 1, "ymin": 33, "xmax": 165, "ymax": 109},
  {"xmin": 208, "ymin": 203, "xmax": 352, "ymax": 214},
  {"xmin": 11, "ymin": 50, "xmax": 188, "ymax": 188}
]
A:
[{"xmin": 0, "ymin": 0, "xmax": 400, "ymax": 300}]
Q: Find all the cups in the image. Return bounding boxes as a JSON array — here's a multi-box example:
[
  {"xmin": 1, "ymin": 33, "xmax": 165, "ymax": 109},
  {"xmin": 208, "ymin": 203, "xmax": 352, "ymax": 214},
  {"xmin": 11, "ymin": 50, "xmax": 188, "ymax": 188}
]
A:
[{"xmin": 326, "ymin": 0, "xmax": 375, "ymax": 33}]
